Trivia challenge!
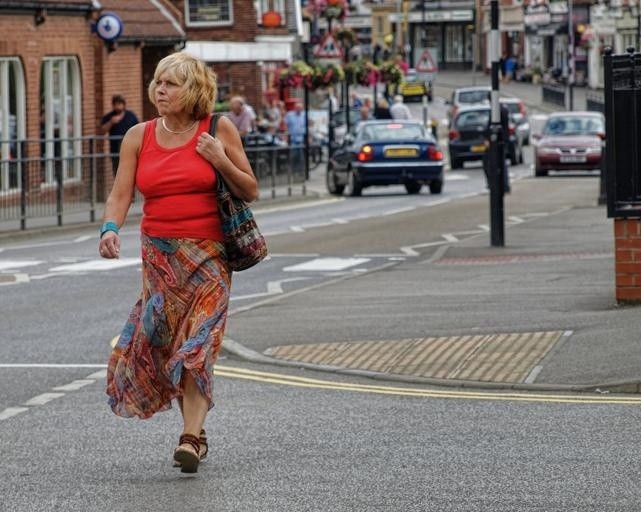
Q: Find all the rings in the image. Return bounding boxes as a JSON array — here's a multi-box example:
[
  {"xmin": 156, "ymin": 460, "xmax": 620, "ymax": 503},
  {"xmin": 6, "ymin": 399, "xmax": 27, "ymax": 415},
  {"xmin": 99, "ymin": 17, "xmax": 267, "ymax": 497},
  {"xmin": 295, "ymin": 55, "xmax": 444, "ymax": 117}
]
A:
[{"xmin": 100, "ymin": 249, "xmax": 104, "ymax": 253}]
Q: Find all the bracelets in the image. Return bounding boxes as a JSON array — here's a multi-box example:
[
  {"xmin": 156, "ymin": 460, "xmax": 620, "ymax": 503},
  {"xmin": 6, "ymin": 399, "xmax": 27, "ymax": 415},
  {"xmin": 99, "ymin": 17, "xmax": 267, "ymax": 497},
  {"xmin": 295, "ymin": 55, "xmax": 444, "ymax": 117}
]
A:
[{"xmin": 99, "ymin": 221, "xmax": 120, "ymax": 239}]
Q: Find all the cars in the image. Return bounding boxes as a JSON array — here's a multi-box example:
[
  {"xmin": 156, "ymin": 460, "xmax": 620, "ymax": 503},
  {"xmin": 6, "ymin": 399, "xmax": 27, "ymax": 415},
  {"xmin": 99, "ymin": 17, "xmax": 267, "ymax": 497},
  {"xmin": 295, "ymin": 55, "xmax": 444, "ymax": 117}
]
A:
[
  {"xmin": 326, "ymin": 116, "xmax": 446, "ymax": 198},
  {"xmin": 446, "ymin": 84, "xmax": 608, "ymax": 179},
  {"xmin": 383, "ymin": 68, "xmax": 434, "ymax": 104}
]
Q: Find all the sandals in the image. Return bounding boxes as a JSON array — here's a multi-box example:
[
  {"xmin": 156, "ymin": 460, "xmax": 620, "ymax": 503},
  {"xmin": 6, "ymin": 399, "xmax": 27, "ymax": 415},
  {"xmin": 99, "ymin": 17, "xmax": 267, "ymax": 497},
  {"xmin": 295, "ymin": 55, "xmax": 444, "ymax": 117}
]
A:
[{"xmin": 172, "ymin": 428, "xmax": 208, "ymax": 475}]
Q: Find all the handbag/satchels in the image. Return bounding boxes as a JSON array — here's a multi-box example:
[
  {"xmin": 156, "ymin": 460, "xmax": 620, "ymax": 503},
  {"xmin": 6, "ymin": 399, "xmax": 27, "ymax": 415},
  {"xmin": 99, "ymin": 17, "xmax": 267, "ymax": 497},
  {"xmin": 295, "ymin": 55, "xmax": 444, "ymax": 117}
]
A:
[{"xmin": 208, "ymin": 113, "xmax": 268, "ymax": 274}]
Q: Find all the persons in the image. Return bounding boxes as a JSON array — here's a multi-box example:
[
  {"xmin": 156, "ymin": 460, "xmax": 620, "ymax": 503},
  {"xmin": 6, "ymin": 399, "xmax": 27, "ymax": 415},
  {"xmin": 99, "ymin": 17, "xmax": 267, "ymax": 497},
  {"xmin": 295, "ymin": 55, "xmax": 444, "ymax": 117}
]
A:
[
  {"xmin": 96, "ymin": 53, "xmax": 259, "ymax": 476},
  {"xmin": 223, "ymin": 92, "xmax": 412, "ymax": 183},
  {"xmin": 500, "ymin": 51, "xmax": 519, "ymax": 85},
  {"xmin": 100, "ymin": 92, "xmax": 142, "ymax": 205}
]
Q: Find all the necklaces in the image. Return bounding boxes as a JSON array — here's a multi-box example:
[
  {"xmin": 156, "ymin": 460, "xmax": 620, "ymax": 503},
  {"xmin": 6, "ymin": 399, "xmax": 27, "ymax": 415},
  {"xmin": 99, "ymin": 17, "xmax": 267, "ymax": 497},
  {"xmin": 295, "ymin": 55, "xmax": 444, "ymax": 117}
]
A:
[{"xmin": 161, "ymin": 117, "xmax": 200, "ymax": 134}]
